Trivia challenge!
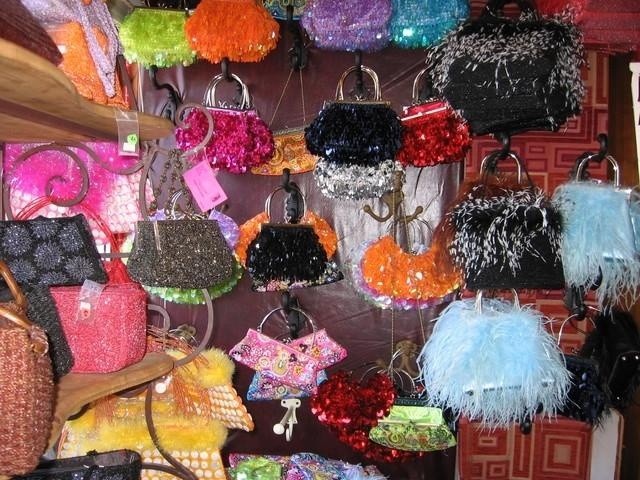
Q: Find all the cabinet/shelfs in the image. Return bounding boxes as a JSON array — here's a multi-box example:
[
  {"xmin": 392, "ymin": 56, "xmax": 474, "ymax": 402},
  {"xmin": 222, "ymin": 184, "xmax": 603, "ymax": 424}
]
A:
[{"xmin": 2, "ymin": 41, "xmax": 179, "ymax": 456}]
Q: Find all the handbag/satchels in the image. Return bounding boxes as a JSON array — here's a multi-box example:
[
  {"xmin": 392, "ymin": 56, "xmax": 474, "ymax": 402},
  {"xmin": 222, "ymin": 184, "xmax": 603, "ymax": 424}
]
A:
[
  {"xmin": 418, "ymin": 286, "xmax": 582, "ymax": 432},
  {"xmin": 389, "ymin": 0, "xmax": 471, "ymax": 50},
  {"xmin": 342, "ymin": 215, "xmax": 465, "ymax": 309},
  {"xmin": 537, "ymin": 313, "xmax": 610, "ymax": 428},
  {"xmin": 119, "ymin": 0, "xmax": 197, "ymax": 68},
  {"xmin": 4, "ymin": 174, "xmax": 254, "ymax": 480},
  {"xmin": 184, "ymin": 0, "xmax": 282, "ymax": 65},
  {"xmin": 392, "ymin": 65, "xmax": 473, "ymax": 168},
  {"xmin": 303, "ymin": 64, "xmax": 405, "ymax": 169},
  {"xmin": 550, "ymin": 153, "xmax": 639, "ymax": 315},
  {"xmin": 228, "ymin": 307, "xmax": 347, "ymax": 400},
  {"xmin": 446, "ymin": 153, "xmax": 570, "ymax": 291},
  {"xmin": 424, "ymin": 0, "xmax": 585, "ymax": 136},
  {"xmin": 312, "ymin": 346, "xmax": 459, "ymax": 465},
  {"xmin": 249, "ymin": 64, "xmax": 327, "ymax": 179},
  {"xmin": 228, "ymin": 451, "xmax": 384, "ymax": 479},
  {"xmin": 580, "ymin": 307, "xmax": 640, "ymax": 414},
  {"xmin": 299, "ymin": 0, "xmax": 394, "ymax": 53},
  {"xmin": 233, "ymin": 185, "xmax": 343, "ymax": 292},
  {"xmin": 173, "ymin": 72, "xmax": 275, "ymax": 176}
]
